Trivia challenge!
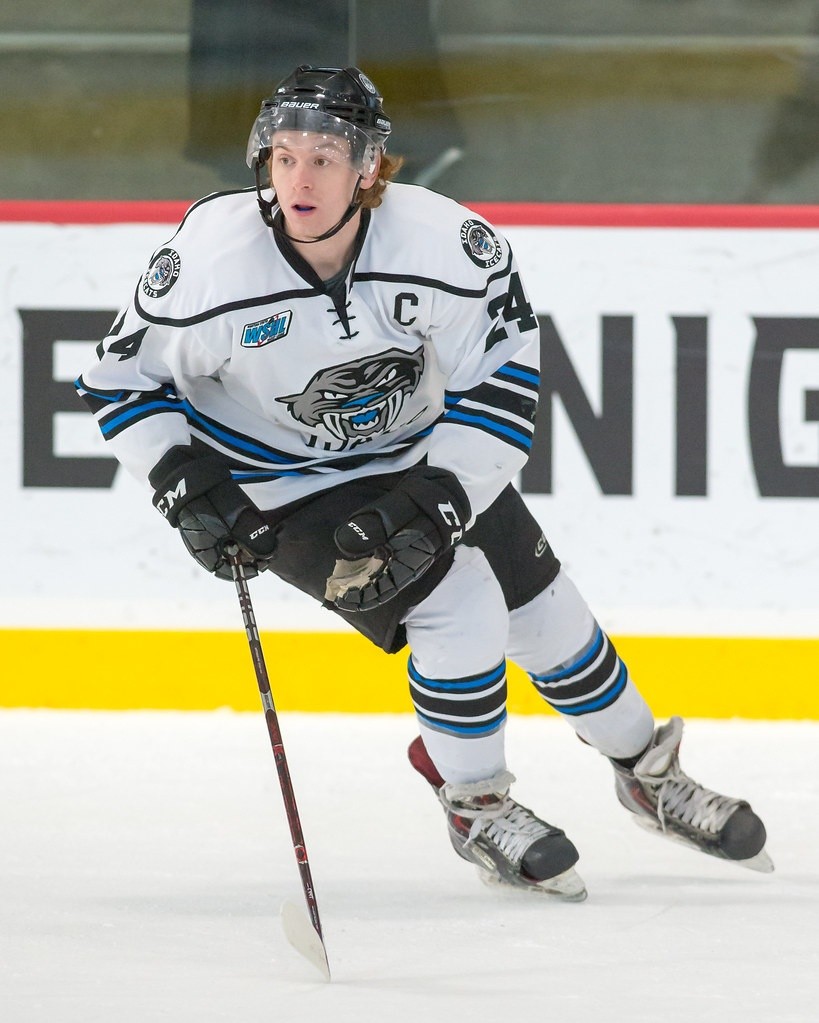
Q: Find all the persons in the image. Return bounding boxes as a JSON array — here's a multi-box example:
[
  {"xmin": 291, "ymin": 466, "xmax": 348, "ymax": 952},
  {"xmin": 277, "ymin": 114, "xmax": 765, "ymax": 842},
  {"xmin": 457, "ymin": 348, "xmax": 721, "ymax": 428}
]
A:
[{"xmin": 75, "ymin": 66, "xmax": 777, "ymax": 902}]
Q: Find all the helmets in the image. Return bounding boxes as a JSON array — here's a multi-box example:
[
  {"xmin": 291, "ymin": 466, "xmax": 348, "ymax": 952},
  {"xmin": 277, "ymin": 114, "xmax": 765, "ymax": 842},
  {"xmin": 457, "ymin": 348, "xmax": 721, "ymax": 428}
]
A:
[{"xmin": 256, "ymin": 64, "xmax": 392, "ymax": 169}]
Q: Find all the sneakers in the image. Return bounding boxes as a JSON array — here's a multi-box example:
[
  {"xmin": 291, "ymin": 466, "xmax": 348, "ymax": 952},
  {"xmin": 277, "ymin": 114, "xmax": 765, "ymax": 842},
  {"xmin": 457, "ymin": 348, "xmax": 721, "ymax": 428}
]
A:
[
  {"xmin": 574, "ymin": 716, "xmax": 774, "ymax": 873},
  {"xmin": 408, "ymin": 735, "xmax": 588, "ymax": 903}
]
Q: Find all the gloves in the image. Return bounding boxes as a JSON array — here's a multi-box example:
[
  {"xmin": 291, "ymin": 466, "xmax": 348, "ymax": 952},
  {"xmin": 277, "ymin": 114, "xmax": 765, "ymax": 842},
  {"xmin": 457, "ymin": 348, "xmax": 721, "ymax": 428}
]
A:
[
  {"xmin": 148, "ymin": 445, "xmax": 286, "ymax": 582},
  {"xmin": 322, "ymin": 465, "xmax": 471, "ymax": 613}
]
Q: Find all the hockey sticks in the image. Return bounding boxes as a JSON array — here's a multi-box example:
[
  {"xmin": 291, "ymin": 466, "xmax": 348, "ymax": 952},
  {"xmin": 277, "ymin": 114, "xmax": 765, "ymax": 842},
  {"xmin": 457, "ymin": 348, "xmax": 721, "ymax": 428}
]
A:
[{"xmin": 225, "ymin": 541, "xmax": 335, "ymax": 980}]
